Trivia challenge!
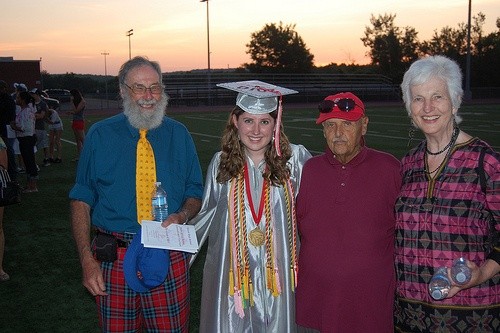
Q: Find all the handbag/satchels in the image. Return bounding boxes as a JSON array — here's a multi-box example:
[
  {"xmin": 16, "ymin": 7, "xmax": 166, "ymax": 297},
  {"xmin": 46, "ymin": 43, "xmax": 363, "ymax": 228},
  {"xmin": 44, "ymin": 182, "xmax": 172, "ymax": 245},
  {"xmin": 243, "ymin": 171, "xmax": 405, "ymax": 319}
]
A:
[{"xmin": 0, "ymin": 164, "xmax": 23, "ymax": 207}]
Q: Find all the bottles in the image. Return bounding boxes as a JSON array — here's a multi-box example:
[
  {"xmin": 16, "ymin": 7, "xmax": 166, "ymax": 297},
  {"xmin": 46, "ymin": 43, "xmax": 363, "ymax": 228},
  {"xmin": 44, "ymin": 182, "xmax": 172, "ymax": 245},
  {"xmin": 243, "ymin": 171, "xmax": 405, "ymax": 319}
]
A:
[
  {"xmin": 151, "ymin": 182, "xmax": 168, "ymax": 222},
  {"xmin": 428, "ymin": 265, "xmax": 452, "ymax": 300},
  {"xmin": 451, "ymin": 257, "xmax": 472, "ymax": 285}
]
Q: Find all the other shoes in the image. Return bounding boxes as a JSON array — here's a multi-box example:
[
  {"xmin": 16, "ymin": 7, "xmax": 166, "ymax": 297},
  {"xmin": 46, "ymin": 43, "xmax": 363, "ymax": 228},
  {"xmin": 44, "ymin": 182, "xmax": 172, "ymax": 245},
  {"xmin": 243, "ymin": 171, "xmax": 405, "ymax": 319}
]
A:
[
  {"xmin": 42, "ymin": 159, "xmax": 51, "ymax": 167},
  {"xmin": 36, "ymin": 165, "xmax": 40, "ymax": 172},
  {"xmin": 55, "ymin": 157, "xmax": 62, "ymax": 163},
  {"xmin": 49, "ymin": 157, "xmax": 56, "ymax": 163},
  {"xmin": 0, "ymin": 272, "xmax": 10, "ymax": 281},
  {"xmin": 16, "ymin": 167, "xmax": 26, "ymax": 174},
  {"xmin": 22, "ymin": 188, "xmax": 39, "ymax": 193}
]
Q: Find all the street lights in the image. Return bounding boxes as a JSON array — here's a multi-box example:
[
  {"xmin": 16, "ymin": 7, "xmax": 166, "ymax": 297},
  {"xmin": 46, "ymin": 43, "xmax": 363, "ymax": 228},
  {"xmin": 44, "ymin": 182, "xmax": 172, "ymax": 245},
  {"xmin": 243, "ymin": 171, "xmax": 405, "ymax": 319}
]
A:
[
  {"xmin": 201, "ymin": 0, "xmax": 210, "ymax": 69},
  {"xmin": 126, "ymin": 29, "xmax": 133, "ymax": 60}
]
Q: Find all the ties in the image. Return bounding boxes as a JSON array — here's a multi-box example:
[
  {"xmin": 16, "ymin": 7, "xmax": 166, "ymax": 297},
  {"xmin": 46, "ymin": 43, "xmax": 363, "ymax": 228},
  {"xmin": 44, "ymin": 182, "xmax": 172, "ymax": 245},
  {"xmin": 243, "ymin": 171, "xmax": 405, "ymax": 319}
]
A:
[{"xmin": 135, "ymin": 128, "xmax": 156, "ymax": 224}]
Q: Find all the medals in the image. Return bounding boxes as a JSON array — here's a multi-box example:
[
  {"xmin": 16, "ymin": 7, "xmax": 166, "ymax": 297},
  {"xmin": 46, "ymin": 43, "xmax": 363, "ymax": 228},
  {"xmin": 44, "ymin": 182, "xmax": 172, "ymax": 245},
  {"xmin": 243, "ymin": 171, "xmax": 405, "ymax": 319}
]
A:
[{"xmin": 249, "ymin": 229, "xmax": 265, "ymax": 246}]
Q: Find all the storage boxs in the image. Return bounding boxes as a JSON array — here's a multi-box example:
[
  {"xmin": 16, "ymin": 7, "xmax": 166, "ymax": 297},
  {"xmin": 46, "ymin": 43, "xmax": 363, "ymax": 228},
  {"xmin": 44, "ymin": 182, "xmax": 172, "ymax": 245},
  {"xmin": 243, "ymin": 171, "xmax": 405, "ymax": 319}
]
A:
[{"xmin": 10, "ymin": 163, "xmax": 41, "ymax": 183}]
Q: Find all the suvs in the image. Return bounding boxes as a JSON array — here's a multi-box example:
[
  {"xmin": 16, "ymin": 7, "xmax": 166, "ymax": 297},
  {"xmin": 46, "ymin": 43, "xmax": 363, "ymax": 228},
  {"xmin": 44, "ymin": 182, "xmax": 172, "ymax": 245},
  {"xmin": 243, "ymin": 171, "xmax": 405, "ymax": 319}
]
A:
[{"xmin": 45, "ymin": 90, "xmax": 71, "ymax": 103}]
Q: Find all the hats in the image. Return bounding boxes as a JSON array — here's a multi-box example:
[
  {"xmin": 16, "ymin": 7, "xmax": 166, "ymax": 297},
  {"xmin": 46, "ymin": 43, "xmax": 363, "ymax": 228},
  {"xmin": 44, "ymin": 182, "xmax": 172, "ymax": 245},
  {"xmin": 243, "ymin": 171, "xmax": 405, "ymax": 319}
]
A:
[
  {"xmin": 15, "ymin": 84, "xmax": 27, "ymax": 90},
  {"xmin": 29, "ymin": 88, "xmax": 41, "ymax": 96},
  {"xmin": 123, "ymin": 227, "xmax": 170, "ymax": 293},
  {"xmin": 316, "ymin": 92, "xmax": 365, "ymax": 124},
  {"xmin": 216, "ymin": 80, "xmax": 299, "ymax": 157}
]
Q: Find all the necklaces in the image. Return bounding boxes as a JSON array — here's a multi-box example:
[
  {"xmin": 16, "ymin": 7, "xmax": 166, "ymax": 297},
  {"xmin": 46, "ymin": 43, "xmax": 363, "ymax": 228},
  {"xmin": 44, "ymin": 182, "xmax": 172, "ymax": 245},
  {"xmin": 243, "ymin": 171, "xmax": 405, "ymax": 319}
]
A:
[{"xmin": 423, "ymin": 127, "xmax": 456, "ymax": 173}]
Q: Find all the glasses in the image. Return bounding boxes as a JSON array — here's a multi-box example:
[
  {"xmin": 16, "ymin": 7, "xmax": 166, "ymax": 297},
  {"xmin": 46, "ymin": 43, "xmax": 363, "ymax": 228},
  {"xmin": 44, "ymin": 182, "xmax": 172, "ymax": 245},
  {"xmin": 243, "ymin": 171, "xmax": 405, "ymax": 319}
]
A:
[
  {"xmin": 318, "ymin": 98, "xmax": 365, "ymax": 115},
  {"xmin": 122, "ymin": 81, "xmax": 165, "ymax": 94}
]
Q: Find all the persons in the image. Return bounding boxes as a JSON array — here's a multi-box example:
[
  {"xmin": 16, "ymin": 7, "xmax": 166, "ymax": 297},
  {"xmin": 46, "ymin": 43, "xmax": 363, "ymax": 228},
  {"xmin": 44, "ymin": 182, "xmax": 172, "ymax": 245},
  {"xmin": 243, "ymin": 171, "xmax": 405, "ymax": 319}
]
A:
[
  {"xmin": 0, "ymin": 80, "xmax": 63, "ymax": 281},
  {"xmin": 69, "ymin": 57, "xmax": 203, "ymax": 333},
  {"xmin": 393, "ymin": 56, "xmax": 500, "ymax": 333},
  {"xmin": 186, "ymin": 80, "xmax": 313, "ymax": 333},
  {"xmin": 66, "ymin": 88, "xmax": 86, "ymax": 160},
  {"xmin": 297, "ymin": 92, "xmax": 402, "ymax": 333}
]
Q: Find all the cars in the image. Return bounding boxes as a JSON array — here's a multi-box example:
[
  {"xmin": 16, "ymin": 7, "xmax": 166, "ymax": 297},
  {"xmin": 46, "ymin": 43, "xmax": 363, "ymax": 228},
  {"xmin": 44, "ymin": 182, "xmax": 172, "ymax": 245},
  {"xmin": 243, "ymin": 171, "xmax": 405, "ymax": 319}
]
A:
[{"xmin": 11, "ymin": 92, "xmax": 60, "ymax": 110}]
